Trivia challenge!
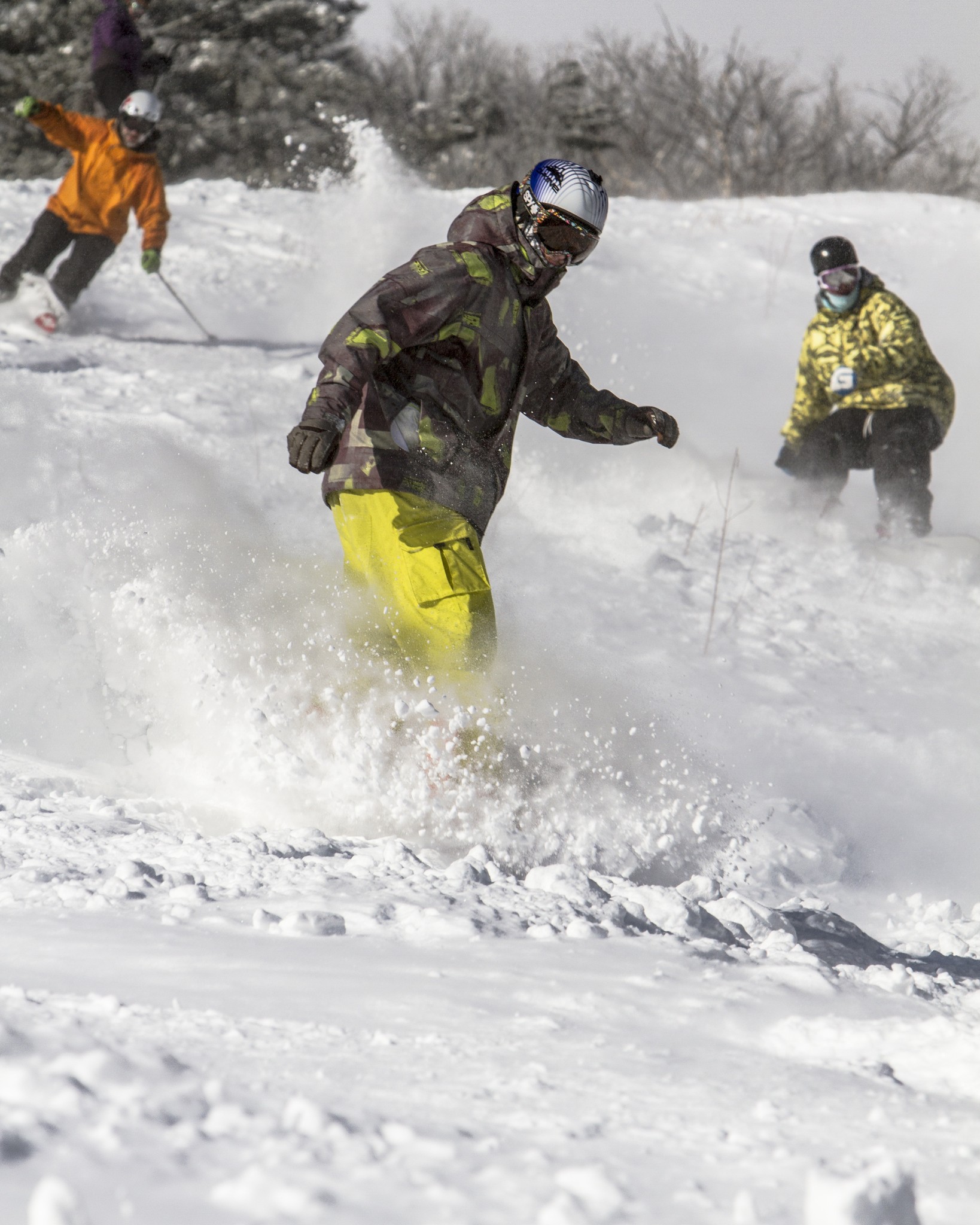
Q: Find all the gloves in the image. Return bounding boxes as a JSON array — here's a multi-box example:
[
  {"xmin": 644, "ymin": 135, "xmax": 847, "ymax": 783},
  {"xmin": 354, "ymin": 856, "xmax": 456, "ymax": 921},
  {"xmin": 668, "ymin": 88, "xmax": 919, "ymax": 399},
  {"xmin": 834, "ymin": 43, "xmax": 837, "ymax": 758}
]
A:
[
  {"xmin": 625, "ymin": 405, "xmax": 679, "ymax": 448},
  {"xmin": 830, "ymin": 365, "xmax": 855, "ymax": 392},
  {"xmin": 141, "ymin": 249, "xmax": 160, "ymax": 274},
  {"xmin": 14, "ymin": 95, "xmax": 40, "ymax": 119},
  {"xmin": 287, "ymin": 418, "xmax": 341, "ymax": 475}
]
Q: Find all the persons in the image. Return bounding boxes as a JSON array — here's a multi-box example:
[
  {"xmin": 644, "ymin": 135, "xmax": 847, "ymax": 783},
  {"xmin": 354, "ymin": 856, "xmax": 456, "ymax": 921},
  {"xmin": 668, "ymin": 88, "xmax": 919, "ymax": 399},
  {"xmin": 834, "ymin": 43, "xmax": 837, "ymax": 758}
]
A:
[
  {"xmin": 285, "ymin": 157, "xmax": 682, "ymax": 785},
  {"xmin": 774, "ymin": 237, "xmax": 958, "ymax": 539},
  {"xmin": 0, "ymin": 0, "xmax": 179, "ymax": 309}
]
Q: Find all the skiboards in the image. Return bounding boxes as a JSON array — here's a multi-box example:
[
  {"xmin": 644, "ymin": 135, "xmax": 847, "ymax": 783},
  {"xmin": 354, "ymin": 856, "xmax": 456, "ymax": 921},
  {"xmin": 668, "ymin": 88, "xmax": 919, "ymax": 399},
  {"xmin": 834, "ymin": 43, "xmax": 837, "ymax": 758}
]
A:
[{"xmin": 0, "ymin": 272, "xmax": 77, "ymax": 338}]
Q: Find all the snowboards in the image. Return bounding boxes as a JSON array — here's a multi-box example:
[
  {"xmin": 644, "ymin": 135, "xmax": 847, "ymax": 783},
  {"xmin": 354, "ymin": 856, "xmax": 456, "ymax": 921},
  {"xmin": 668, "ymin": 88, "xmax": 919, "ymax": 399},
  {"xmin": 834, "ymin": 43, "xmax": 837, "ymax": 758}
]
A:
[{"xmin": 843, "ymin": 534, "xmax": 980, "ymax": 583}]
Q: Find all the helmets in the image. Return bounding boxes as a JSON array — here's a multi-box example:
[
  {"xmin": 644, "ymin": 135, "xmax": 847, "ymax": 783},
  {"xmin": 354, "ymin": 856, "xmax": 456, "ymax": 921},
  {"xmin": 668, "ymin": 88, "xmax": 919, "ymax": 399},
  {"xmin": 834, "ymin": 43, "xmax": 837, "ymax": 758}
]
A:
[
  {"xmin": 518, "ymin": 159, "xmax": 609, "ymax": 237},
  {"xmin": 119, "ymin": 90, "xmax": 163, "ymax": 124},
  {"xmin": 810, "ymin": 236, "xmax": 860, "ymax": 277}
]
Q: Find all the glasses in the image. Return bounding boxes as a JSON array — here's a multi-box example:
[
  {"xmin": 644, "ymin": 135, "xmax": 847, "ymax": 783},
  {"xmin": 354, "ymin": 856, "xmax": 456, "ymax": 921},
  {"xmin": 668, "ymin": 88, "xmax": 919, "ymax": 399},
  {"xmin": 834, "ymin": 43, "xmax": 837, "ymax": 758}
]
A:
[
  {"xmin": 121, "ymin": 113, "xmax": 155, "ymax": 134},
  {"xmin": 525, "ymin": 209, "xmax": 600, "ymax": 266},
  {"xmin": 816, "ymin": 264, "xmax": 860, "ymax": 296}
]
